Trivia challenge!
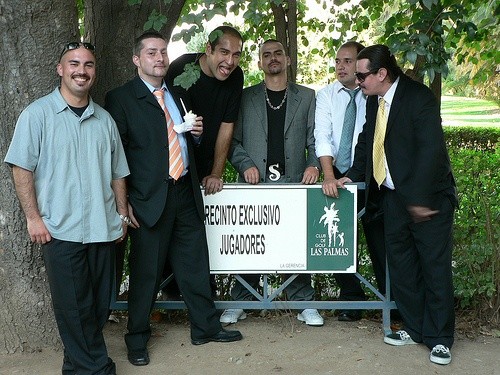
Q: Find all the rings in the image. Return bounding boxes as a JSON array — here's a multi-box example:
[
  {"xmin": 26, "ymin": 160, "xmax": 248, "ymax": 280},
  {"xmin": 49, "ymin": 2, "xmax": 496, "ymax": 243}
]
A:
[{"xmin": 120, "ymin": 237, "xmax": 123, "ymax": 241}]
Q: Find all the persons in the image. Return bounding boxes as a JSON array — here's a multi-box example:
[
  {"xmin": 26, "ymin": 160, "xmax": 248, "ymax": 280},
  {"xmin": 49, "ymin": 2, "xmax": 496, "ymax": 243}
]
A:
[
  {"xmin": 220, "ymin": 39, "xmax": 323, "ymax": 323},
  {"xmin": 314, "ymin": 41, "xmax": 368, "ymax": 199},
  {"xmin": 165, "ymin": 26, "xmax": 244, "ymax": 196},
  {"xmin": 13, "ymin": 42, "xmax": 131, "ymax": 375},
  {"xmin": 338, "ymin": 44, "xmax": 459, "ymax": 365},
  {"xmin": 104, "ymin": 31, "xmax": 243, "ymax": 366}
]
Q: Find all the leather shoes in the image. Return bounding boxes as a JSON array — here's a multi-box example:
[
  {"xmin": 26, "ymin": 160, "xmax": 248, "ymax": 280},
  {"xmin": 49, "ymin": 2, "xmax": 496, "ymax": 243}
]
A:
[
  {"xmin": 338, "ymin": 310, "xmax": 362, "ymax": 321},
  {"xmin": 193, "ymin": 329, "xmax": 243, "ymax": 345},
  {"xmin": 128, "ymin": 349, "xmax": 150, "ymax": 365}
]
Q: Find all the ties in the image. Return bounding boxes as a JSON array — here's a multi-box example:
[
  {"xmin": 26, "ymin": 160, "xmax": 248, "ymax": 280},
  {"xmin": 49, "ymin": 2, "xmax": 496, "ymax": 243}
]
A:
[
  {"xmin": 335, "ymin": 87, "xmax": 361, "ymax": 174},
  {"xmin": 373, "ymin": 98, "xmax": 387, "ymax": 187},
  {"xmin": 153, "ymin": 89, "xmax": 184, "ymax": 181}
]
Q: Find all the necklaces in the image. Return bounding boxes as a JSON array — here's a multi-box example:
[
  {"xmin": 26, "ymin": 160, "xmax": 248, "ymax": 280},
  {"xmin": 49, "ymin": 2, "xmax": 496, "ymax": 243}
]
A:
[{"xmin": 265, "ymin": 86, "xmax": 288, "ymax": 110}]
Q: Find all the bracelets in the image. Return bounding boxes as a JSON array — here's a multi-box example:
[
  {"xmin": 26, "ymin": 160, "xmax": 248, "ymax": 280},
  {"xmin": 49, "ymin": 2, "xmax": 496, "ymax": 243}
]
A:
[
  {"xmin": 119, "ymin": 214, "xmax": 131, "ymax": 226},
  {"xmin": 313, "ymin": 166, "xmax": 320, "ymax": 172}
]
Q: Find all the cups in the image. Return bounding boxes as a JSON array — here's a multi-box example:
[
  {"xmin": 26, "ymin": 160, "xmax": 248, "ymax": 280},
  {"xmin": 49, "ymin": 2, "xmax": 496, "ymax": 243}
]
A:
[{"xmin": 183, "ymin": 114, "xmax": 197, "ymax": 123}]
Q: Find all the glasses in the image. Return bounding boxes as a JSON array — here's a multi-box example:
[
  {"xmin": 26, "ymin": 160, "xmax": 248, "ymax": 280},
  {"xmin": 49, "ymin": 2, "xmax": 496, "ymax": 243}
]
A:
[
  {"xmin": 354, "ymin": 70, "xmax": 378, "ymax": 83},
  {"xmin": 59, "ymin": 42, "xmax": 95, "ymax": 63}
]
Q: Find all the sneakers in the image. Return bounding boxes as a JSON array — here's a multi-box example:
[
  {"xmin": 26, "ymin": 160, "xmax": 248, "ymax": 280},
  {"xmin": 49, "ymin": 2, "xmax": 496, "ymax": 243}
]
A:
[
  {"xmin": 298, "ymin": 309, "xmax": 324, "ymax": 325},
  {"xmin": 383, "ymin": 330, "xmax": 419, "ymax": 345},
  {"xmin": 429, "ymin": 344, "xmax": 450, "ymax": 365},
  {"xmin": 219, "ymin": 308, "xmax": 247, "ymax": 323}
]
